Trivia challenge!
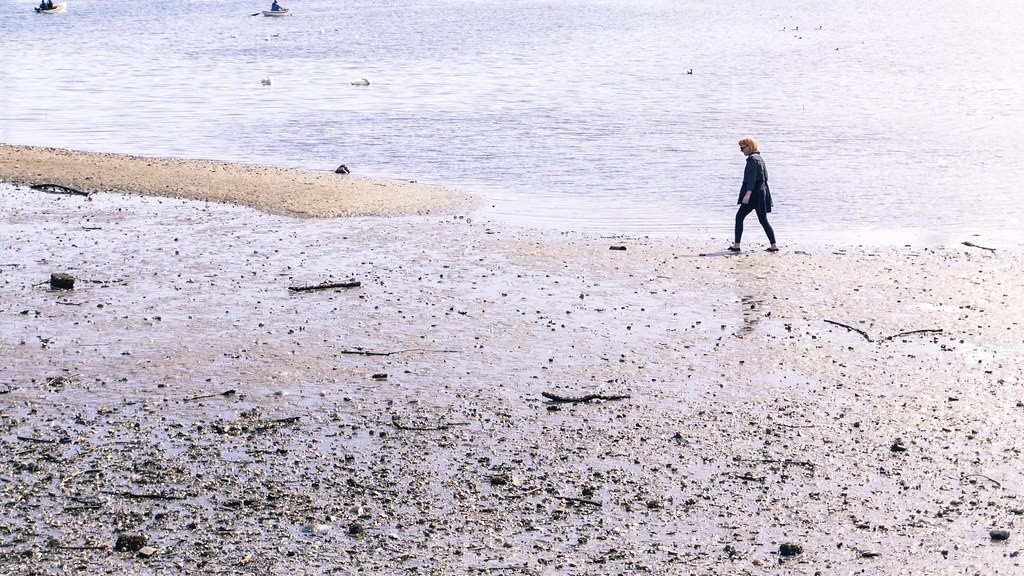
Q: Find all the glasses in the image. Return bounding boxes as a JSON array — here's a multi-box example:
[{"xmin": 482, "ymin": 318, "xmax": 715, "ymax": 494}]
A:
[{"xmin": 741, "ymin": 145, "xmax": 748, "ymax": 151}]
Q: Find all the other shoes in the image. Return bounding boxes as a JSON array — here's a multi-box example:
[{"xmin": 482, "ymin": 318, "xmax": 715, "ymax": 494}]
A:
[
  {"xmin": 765, "ymin": 248, "xmax": 780, "ymax": 252},
  {"xmin": 728, "ymin": 246, "xmax": 740, "ymax": 252}
]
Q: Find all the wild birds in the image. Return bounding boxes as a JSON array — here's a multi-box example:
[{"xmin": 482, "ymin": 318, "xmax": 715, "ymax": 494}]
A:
[
  {"xmin": 320, "ymin": 27, "xmax": 325, "ymax": 33},
  {"xmin": 335, "ymin": 28, "xmax": 340, "ymax": 32},
  {"xmin": 836, "ymin": 48, "xmax": 838, "ymax": 50},
  {"xmin": 231, "ymin": 33, "xmax": 237, "ymax": 38},
  {"xmin": 265, "ymin": 36, "xmax": 270, "ymax": 41},
  {"xmin": 779, "ymin": 26, "xmax": 822, "ymax": 39},
  {"xmin": 687, "ymin": 69, "xmax": 692, "ymax": 74},
  {"xmin": 351, "ymin": 78, "xmax": 370, "ymax": 86},
  {"xmin": 261, "ymin": 77, "xmax": 272, "ymax": 85},
  {"xmin": 273, "ymin": 33, "xmax": 279, "ymax": 37}
]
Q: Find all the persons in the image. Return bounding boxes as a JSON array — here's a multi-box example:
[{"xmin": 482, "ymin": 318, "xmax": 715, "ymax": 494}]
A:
[
  {"xmin": 271, "ymin": 0, "xmax": 283, "ymax": 11},
  {"xmin": 40, "ymin": 0, "xmax": 53, "ymax": 10},
  {"xmin": 728, "ymin": 137, "xmax": 779, "ymax": 253}
]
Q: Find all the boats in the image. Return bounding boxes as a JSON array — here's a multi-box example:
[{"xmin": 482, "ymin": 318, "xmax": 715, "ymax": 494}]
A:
[
  {"xmin": 34, "ymin": 2, "xmax": 66, "ymax": 14},
  {"xmin": 262, "ymin": 8, "xmax": 292, "ymax": 16}
]
